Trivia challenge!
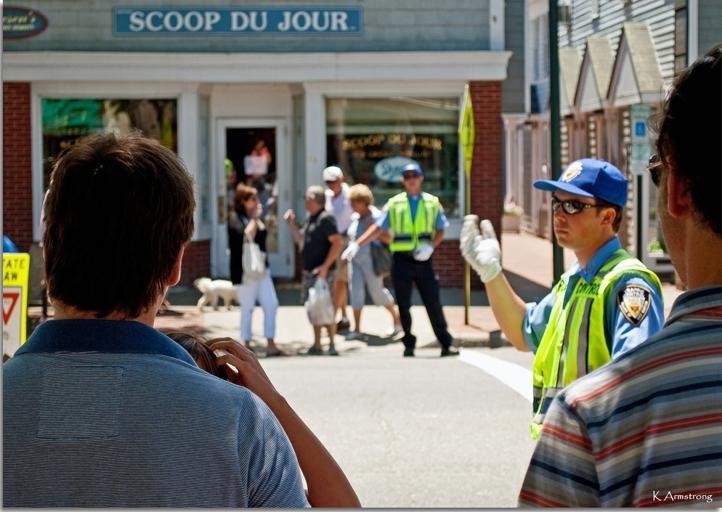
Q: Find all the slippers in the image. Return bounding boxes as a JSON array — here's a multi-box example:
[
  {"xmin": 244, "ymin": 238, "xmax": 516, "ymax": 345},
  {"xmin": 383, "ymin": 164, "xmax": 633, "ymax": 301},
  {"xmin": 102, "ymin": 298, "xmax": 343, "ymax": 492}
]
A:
[{"xmin": 265, "ymin": 350, "xmax": 286, "ymax": 356}]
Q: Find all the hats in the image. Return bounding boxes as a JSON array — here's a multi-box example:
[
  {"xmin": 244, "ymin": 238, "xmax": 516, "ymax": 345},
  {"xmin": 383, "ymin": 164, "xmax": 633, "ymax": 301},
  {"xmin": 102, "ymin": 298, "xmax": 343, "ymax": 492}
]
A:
[
  {"xmin": 533, "ymin": 158, "xmax": 627, "ymax": 208},
  {"xmin": 322, "ymin": 166, "xmax": 342, "ymax": 181},
  {"xmin": 402, "ymin": 163, "xmax": 424, "ymax": 176}
]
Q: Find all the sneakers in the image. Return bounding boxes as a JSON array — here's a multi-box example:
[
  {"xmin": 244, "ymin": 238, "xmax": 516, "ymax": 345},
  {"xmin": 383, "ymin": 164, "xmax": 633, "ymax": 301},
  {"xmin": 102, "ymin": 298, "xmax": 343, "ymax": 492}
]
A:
[
  {"xmin": 391, "ymin": 325, "xmax": 403, "ymax": 336},
  {"xmin": 308, "ymin": 319, "xmax": 361, "ymax": 355}
]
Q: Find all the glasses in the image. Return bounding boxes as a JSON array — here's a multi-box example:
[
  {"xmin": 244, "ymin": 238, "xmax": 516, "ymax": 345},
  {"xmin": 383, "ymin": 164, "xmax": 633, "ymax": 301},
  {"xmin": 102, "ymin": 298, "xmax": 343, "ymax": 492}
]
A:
[
  {"xmin": 648, "ymin": 154, "xmax": 667, "ymax": 187},
  {"xmin": 551, "ymin": 195, "xmax": 605, "ymax": 215}
]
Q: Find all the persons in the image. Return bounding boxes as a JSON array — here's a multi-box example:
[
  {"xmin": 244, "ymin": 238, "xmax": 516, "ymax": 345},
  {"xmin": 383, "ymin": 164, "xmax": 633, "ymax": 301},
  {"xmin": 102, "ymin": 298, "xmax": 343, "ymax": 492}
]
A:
[
  {"xmin": 517, "ymin": 42, "xmax": 721, "ymax": 511},
  {"xmin": 3, "ymin": 131, "xmax": 309, "ymax": 507},
  {"xmin": 457, "ymin": 158, "xmax": 664, "ymax": 444},
  {"xmin": 159, "ymin": 323, "xmax": 363, "ymax": 508},
  {"xmin": 224, "ymin": 136, "xmax": 458, "ymax": 360}
]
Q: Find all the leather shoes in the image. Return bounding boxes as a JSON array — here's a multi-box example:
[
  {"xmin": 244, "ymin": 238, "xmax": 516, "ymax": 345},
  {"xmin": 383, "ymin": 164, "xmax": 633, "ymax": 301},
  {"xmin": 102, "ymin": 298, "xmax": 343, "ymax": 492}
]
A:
[
  {"xmin": 441, "ymin": 345, "xmax": 459, "ymax": 356},
  {"xmin": 403, "ymin": 348, "xmax": 414, "ymax": 356}
]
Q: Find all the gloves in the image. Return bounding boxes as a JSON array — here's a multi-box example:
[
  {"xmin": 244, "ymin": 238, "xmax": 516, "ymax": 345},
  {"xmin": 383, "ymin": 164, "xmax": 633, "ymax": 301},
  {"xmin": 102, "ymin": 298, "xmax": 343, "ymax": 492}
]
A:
[
  {"xmin": 459, "ymin": 214, "xmax": 502, "ymax": 283},
  {"xmin": 414, "ymin": 241, "xmax": 436, "ymax": 261},
  {"xmin": 340, "ymin": 240, "xmax": 361, "ymax": 260}
]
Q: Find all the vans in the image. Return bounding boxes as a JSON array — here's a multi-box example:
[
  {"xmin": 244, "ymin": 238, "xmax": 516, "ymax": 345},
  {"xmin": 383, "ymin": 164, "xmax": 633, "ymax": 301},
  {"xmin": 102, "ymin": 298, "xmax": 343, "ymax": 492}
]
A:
[{"xmin": 369, "ymin": 169, "xmax": 447, "ymax": 210}]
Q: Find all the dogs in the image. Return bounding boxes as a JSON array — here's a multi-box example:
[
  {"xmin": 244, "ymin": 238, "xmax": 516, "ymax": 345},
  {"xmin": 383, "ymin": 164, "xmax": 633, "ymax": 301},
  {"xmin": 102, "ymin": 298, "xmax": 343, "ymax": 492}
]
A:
[{"xmin": 192, "ymin": 277, "xmax": 244, "ymax": 313}]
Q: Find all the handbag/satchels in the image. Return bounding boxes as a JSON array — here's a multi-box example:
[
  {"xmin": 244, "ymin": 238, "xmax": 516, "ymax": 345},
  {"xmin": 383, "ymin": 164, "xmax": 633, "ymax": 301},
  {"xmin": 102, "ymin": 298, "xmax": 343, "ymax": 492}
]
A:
[
  {"xmin": 370, "ymin": 241, "xmax": 393, "ymax": 275},
  {"xmin": 242, "ymin": 230, "xmax": 267, "ymax": 283}
]
